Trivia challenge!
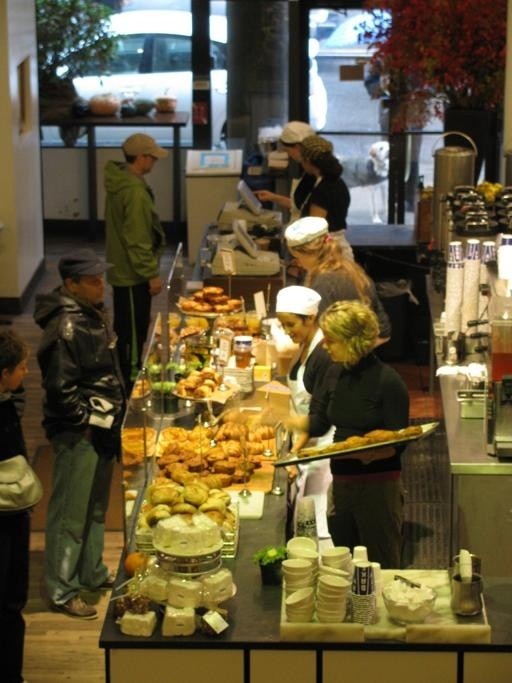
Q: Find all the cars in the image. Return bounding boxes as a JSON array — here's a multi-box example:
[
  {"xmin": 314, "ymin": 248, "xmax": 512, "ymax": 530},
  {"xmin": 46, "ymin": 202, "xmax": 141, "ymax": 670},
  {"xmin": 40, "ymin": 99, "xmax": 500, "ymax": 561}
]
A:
[
  {"xmin": 309, "ymin": 11, "xmax": 347, "ymax": 40},
  {"xmin": 40, "ymin": 8, "xmax": 328, "ymax": 146}
]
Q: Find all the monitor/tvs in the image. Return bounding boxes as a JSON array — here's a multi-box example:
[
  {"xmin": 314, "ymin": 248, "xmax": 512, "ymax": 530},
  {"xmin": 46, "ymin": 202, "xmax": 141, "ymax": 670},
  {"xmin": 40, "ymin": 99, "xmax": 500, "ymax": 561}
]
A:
[
  {"xmin": 238, "ymin": 180, "xmax": 262, "ymax": 214},
  {"xmin": 233, "ymin": 220, "xmax": 257, "ymax": 258}
]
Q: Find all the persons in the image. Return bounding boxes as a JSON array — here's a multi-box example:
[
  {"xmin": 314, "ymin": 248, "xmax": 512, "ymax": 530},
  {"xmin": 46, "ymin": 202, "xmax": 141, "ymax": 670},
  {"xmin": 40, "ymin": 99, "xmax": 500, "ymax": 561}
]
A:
[
  {"xmin": 0, "ymin": 330, "xmax": 33, "ymax": 682},
  {"xmin": 103, "ymin": 133, "xmax": 169, "ymax": 373},
  {"xmin": 254, "ymin": 121, "xmax": 409, "ymax": 570},
  {"xmin": 32, "ymin": 246, "xmax": 128, "ymax": 620}
]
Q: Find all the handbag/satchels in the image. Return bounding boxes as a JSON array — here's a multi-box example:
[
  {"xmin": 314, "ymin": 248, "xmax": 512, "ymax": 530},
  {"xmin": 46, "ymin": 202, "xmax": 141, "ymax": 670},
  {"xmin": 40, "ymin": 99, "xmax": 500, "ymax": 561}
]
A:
[{"xmin": 0, "ymin": 454, "xmax": 45, "ymax": 512}]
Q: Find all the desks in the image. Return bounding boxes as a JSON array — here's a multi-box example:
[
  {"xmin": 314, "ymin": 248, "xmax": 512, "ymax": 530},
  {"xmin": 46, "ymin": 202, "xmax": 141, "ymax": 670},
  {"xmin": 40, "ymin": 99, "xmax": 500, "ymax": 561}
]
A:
[{"xmin": 41, "ymin": 111, "xmax": 189, "ymax": 249}]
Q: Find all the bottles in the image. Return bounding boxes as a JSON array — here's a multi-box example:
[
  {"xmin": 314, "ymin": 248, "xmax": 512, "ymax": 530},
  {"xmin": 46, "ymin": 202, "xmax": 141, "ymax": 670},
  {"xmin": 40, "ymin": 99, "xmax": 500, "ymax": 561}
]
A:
[{"xmin": 452, "ymin": 574, "xmax": 484, "ymax": 617}]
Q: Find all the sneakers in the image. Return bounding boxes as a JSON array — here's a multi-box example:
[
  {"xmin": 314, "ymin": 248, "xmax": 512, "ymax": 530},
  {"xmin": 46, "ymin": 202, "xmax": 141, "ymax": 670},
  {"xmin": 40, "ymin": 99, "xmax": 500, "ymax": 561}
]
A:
[
  {"xmin": 97, "ymin": 573, "xmax": 116, "ymax": 591},
  {"xmin": 60, "ymin": 594, "xmax": 98, "ymax": 621}
]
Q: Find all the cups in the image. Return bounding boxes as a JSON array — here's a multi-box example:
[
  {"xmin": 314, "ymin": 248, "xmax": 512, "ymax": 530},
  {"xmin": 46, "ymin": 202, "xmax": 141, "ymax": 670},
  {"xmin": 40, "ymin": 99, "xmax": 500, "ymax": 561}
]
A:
[
  {"xmin": 461, "ymin": 239, "xmax": 480, "ymax": 332},
  {"xmin": 234, "ymin": 337, "xmax": 252, "ymax": 369},
  {"xmin": 444, "ymin": 241, "xmax": 463, "ymax": 332},
  {"xmin": 295, "ymin": 499, "xmax": 315, "ymax": 541},
  {"xmin": 477, "ymin": 241, "xmax": 495, "ymax": 329},
  {"xmin": 351, "ymin": 547, "xmax": 379, "ymax": 626},
  {"xmin": 453, "ymin": 553, "xmax": 482, "ymax": 576}
]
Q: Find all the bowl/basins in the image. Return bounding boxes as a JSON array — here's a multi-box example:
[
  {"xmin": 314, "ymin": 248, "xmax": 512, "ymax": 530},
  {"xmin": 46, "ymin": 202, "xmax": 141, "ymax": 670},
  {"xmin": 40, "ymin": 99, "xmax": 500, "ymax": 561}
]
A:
[
  {"xmin": 315, "ymin": 577, "xmax": 350, "ymax": 625},
  {"xmin": 321, "ymin": 547, "xmax": 351, "ymax": 574},
  {"xmin": 381, "ymin": 584, "xmax": 440, "ymax": 624},
  {"xmin": 284, "ymin": 535, "xmax": 319, "ymax": 597},
  {"xmin": 285, "ymin": 589, "xmax": 315, "ymax": 625}
]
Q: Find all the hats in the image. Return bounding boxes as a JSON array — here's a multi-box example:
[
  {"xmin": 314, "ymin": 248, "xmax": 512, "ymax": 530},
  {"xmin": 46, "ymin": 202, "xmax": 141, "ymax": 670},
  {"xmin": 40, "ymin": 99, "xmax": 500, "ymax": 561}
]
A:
[
  {"xmin": 284, "ymin": 214, "xmax": 328, "ymax": 248},
  {"xmin": 275, "ymin": 282, "xmax": 322, "ymax": 319},
  {"xmin": 57, "ymin": 247, "xmax": 115, "ymax": 278},
  {"xmin": 299, "ymin": 136, "xmax": 334, "ymax": 168},
  {"xmin": 280, "ymin": 118, "xmax": 317, "ymax": 146},
  {"xmin": 122, "ymin": 132, "xmax": 172, "ymax": 163}
]
